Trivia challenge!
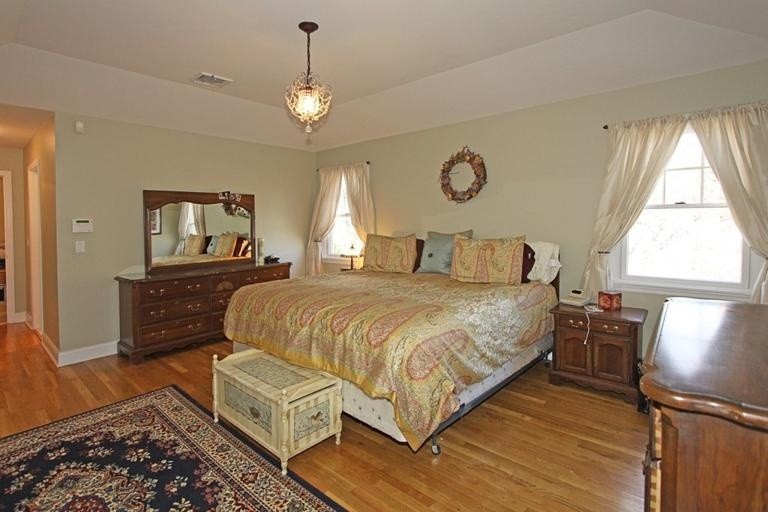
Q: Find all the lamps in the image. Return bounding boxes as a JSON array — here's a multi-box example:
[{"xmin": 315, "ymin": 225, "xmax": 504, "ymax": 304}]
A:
[
  {"xmin": 284, "ymin": 21, "xmax": 333, "ymax": 133},
  {"xmin": 340, "ymin": 244, "xmax": 363, "ymax": 271}
]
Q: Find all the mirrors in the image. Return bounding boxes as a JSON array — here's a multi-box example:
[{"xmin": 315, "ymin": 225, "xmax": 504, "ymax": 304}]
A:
[{"xmin": 151, "ymin": 202, "xmax": 251, "ymax": 265}]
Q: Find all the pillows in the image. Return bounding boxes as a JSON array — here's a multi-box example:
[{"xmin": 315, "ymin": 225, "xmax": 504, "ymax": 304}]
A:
[
  {"xmin": 518, "ymin": 244, "xmax": 535, "ymax": 282},
  {"xmin": 413, "ymin": 239, "xmax": 424, "ymax": 273},
  {"xmin": 202, "ymin": 235, "xmax": 213, "ymax": 254},
  {"xmin": 233, "ymin": 236, "xmax": 251, "ymax": 257},
  {"xmin": 183, "ymin": 234, "xmax": 205, "ymax": 255},
  {"xmin": 363, "ymin": 233, "xmax": 417, "ymax": 274},
  {"xmin": 207, "ymin": 235, "xmax": 219, "ymax": 254},
  {"xmin": 526, "ymin": 242, "xmax": 562, "ymax": 286},
  {"xmin": 415, "ymin": 229, "xmax": 473, "ymax": 274},
  {"xmin": 449, "ymin": 234, "xmax": 525, "ymax": 287},
  {"xmin": 175, "ymin": 240, "xmax": 184, "ymax": 255},
  {"xmin": 213, "ymin": 232, "xmax": 238, "ymax": 256}
]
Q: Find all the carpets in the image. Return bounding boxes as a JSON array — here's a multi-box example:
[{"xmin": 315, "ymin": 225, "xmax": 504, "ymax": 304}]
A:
[{"xmin": 0, "ymin": 382, "xmax": 349, "ymax": 512}]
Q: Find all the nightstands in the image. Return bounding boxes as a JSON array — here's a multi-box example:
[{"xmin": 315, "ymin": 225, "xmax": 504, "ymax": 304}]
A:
[{"xmin": 549, "ymin": 302, "xmax": 648, "ymax": 406}]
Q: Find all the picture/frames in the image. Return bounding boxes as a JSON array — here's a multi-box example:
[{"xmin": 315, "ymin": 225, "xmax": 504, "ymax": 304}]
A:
[{"xmin": 152, "ymin": 207, "xmax": 162, "ymax": 235}]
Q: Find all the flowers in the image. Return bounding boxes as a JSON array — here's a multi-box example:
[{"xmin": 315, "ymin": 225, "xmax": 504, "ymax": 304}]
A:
[{"xmin": 438, "ymin": 144, "xmax": 488, "ymax": 204}]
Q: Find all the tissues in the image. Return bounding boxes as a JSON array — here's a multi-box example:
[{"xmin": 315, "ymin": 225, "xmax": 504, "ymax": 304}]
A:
[{"xmin": 598, "ymin": 290, "xmax": 622, "ymax": 311}]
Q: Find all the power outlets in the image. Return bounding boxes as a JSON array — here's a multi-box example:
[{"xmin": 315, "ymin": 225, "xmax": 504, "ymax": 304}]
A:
[{"xmin": 76, "ymin": 241, "xmax": 85, "ymax": 253}]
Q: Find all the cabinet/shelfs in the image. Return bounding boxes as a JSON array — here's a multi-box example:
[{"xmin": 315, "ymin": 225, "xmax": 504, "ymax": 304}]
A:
[
  {"xmin": 212, "ymin": 349, "xmax": 342, "ymax": 476},
  {"xmin": 639, "ymin": 297, "xmax": 768, "ymax": 512},
  {"xmin": 114, "ymin": 258, "xmax": 293, "ymax": 365}
]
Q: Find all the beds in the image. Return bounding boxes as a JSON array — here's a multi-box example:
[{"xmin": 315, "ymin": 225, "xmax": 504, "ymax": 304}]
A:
[
  {"xmin": 152, "ymin": 231, "xmax": 251, "ymax": 266},
  {"xmin": 224, "ymin": 230, "xmax": 561, "ymax": 455}
]
